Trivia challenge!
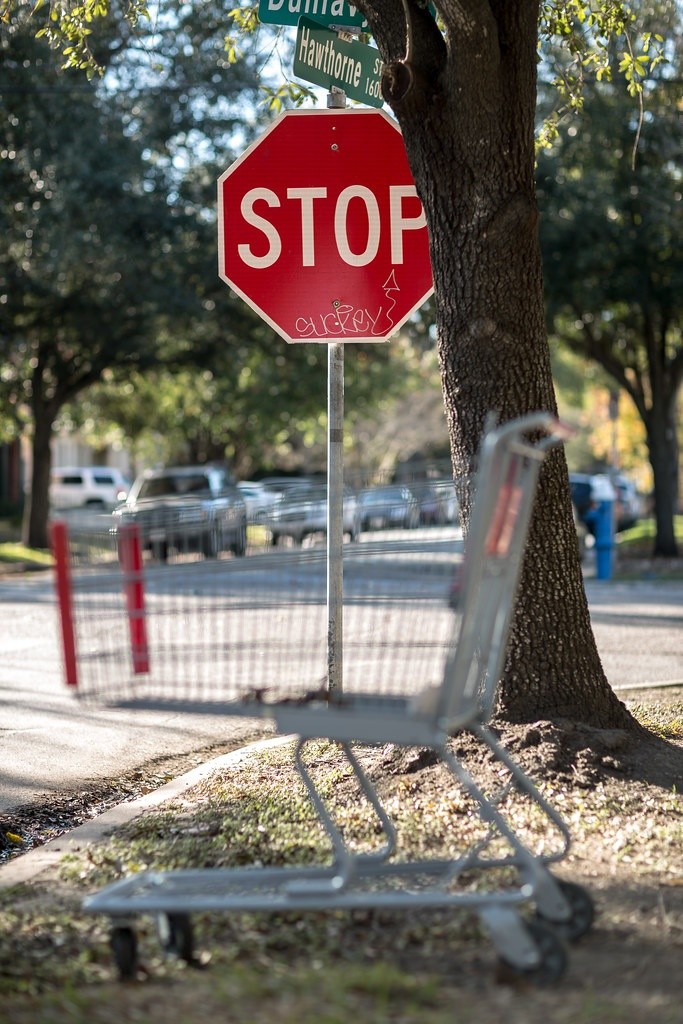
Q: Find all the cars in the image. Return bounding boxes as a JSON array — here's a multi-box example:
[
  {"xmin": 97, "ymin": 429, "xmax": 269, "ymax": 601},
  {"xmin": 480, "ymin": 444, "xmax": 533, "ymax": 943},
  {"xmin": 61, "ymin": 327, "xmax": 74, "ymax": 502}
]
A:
[
  {"xmin": 236, "ymin": 459, "xmax": 461, "ymax": 551},
  {"xmin": 51, "ymin": 462, "xmax": 131, "ymax": 512},
  {"xmin": 113, "ymin": 461, "xmax": 245, "ymax": 566}
]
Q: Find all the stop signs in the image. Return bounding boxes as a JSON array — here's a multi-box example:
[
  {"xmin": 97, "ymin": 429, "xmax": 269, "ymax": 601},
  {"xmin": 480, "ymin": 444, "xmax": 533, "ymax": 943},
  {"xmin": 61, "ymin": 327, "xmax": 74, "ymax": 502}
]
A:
[{"xmin": 215, "ymin": 109, "xmax": 435, "ymax": 345}]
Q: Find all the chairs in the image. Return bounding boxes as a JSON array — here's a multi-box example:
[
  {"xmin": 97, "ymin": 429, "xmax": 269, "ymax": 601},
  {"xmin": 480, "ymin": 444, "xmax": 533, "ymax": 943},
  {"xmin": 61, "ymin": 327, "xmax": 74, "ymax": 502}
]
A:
[
  {"xmin": 145, "ymin": 479, "xmax": 174, "ymax": 497},
  {"xmin": 186, "ymin": 477, "xmax": 210, "ymax": 494}
]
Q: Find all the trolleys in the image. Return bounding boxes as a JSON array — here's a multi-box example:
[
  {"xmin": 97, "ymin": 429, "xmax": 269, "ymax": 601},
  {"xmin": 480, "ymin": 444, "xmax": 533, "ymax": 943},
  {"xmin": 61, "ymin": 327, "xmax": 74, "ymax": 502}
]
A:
[{"xmin": 52, "ymin": 411, "xmax": 596, "ymax": 981}]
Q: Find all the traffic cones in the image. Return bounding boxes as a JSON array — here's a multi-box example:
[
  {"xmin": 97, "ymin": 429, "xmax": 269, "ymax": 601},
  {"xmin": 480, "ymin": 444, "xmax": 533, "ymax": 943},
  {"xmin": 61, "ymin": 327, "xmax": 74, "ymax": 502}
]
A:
[
  {"xmin": 109, "ymin": 520, "xmax": 151, "ymax": 677},
  {"xmin": 46, "ymin": 516, "xmax": 81, "ymax": 686}
]
